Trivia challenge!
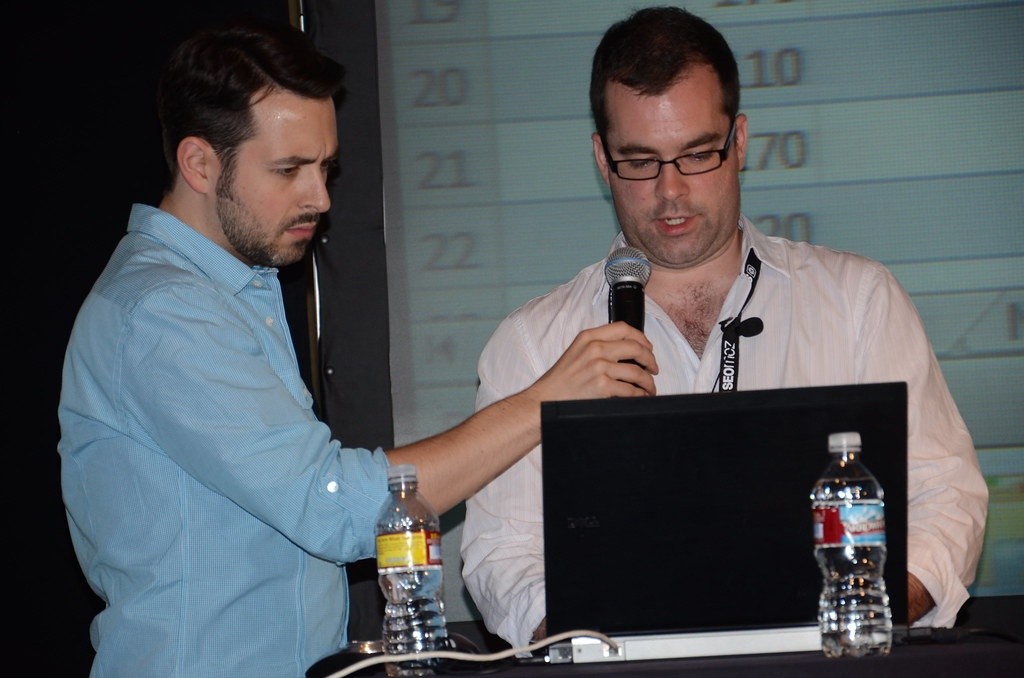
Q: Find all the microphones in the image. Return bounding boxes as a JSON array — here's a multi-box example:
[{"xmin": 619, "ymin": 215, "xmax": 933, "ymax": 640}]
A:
[
  {"xmin": 603, "ymin": 247, "xmax": 653, "ymax": 389},
  {"xmin": 711, "ymin": 317, "xmax": 764, "ymax": 393}
]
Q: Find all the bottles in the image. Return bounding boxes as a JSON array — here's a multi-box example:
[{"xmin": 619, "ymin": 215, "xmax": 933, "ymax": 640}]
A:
[
  {"xmin": 375, "ymin": 463, "xmax": 451, "ymax": 667},
  {"xmin": 807, "ymin": 430, "xmax": 892, "ymax": 658}
]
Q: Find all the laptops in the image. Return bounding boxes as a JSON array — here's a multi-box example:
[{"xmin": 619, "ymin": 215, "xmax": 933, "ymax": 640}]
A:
[{"xmin": 539, "ymin": 381, "xmax": 912, "ymax": 664}]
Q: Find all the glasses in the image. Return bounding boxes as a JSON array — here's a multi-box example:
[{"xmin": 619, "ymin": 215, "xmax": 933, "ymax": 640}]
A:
[{"xmin": 600, "ymin": 116, "xmax": 736, "ymax": 181}]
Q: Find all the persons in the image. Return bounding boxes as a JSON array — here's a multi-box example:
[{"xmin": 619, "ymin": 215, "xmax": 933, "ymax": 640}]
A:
[
  {"xmin": 56, "ymin": 20, "xmax": 658, "ymax": 678},
  {"xmin": 460, "ymin": 5, "xmax": 989, "ymax": 658}
]
